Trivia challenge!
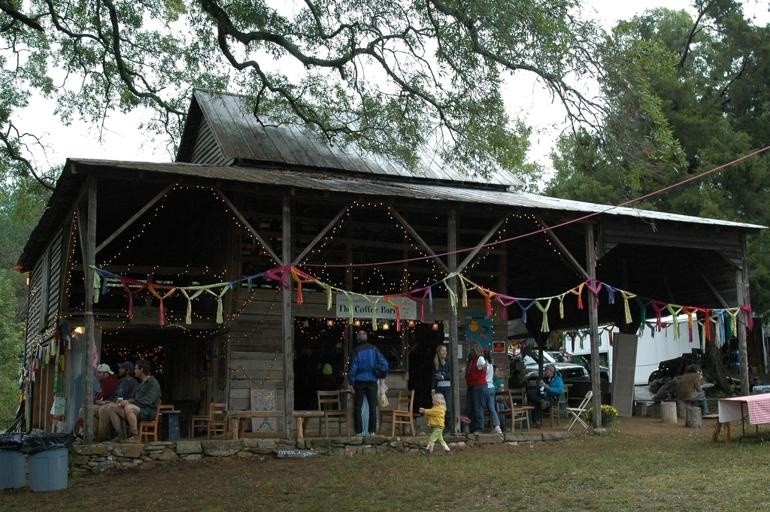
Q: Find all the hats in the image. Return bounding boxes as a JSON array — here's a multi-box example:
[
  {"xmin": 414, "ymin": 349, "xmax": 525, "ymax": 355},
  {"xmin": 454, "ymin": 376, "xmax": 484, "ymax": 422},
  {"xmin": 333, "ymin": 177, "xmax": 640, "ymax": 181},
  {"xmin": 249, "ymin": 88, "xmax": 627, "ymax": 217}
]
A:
[{"xmin": 98, "ymin": 362, "xmax": 115, "ymax": 376}]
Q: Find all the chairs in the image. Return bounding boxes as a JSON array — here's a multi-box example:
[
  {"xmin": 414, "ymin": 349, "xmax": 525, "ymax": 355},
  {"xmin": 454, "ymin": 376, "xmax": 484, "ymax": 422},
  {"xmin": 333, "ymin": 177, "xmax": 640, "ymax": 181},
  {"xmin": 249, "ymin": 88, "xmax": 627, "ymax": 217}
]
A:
[
  {"xmin": 494, "ymin": 380, "xmax": 593, "ymax": 432},
  {"xmin": 378, "ymin": 389, "xmax": 415, "ymax": 438},
  {"xmin": 121, "ymin": 399, "xmax": 161, "ymax": 443},
  {"xmin": 208, "ymin": 400, "xmax": 227, "ymax": 440},
  {"xmin": 317, "ymin": 390, "xmax": 350, "ymax": 438}
]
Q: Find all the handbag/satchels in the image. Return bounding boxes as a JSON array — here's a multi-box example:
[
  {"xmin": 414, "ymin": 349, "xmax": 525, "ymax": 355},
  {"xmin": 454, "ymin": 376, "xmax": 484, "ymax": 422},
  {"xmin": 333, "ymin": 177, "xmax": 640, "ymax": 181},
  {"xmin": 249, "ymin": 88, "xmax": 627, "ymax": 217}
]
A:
[{"xmin": 374, "ymin": 362, "xmax": 387, "ymax": 379}]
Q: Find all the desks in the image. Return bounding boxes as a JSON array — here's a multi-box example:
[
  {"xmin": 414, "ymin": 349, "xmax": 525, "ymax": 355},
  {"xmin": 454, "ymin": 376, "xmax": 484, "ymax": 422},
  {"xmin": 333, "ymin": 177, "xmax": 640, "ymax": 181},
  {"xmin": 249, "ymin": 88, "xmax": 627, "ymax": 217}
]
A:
[
  {"xmin": 188, "ymin": 417, "xmax": 208, "ymax": 440},
  {"xmin": 717, "ymin": 393, "xmax": 770, "ymax": 442}
]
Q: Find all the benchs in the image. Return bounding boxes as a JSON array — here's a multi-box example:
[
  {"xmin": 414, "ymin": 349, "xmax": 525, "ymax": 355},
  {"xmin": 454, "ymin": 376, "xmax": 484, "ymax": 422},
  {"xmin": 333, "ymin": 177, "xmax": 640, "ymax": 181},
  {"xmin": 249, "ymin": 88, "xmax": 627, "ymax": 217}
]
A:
[
  {"xmin": 633, "ymin": 399, "xmax": 705, "ymax": 427},
  {"xmin": 702, "ymin": 414, "xmax": 730, "ymax": 442},
  {"xmin": 228, "ymin": 409, "xmax": 324, "ymax": 440}
]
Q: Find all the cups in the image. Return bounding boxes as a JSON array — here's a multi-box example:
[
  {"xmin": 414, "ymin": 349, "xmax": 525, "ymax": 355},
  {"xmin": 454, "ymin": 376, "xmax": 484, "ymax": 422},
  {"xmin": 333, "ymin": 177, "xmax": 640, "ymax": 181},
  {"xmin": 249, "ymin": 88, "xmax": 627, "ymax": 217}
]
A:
[
  {"xmin": 118, "ymin": 397, "xmax": 124, "ymax": 403},
  {"xmin": 499, "ymin": 384, "xmax": 504, "ymax": 391}
]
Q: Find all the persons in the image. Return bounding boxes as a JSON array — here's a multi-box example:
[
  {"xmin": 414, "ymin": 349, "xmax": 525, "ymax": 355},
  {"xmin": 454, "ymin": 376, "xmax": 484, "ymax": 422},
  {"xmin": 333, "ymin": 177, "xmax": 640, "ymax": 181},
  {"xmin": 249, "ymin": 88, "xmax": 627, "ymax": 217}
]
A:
[
  {"xmin": 418, "ymin": 392, "xmax": 455, "ymax": 456},
  {"xmin": 675, "ymin": 363, "xmax": 720, "ymax": 418},
  {"xmin": 493, "ymin": 365, "xmax": 505, "ymax": 392},
  {"xmin": 76, "ymin": 361, "xmax": 140, "ymax": 442},
  {"xmin": 349, "ymin": 330, "xmax": 388, "ymax": 438},
  {"xmin": 539, "ymin": 365, "xmax": 565, "ymax": 401},
  {"xmin": 432, "ymin": 343, "xmax": 454, "ymax": 433},
  {"xmin": 107, "ymin": 358, "xmax": 161, "ymax": 444},
  {"xmin": 458, "ymin": 339, "xmax": 488, "ymax": 434},
  {"xmin": 319, "ymin": 362, "xmax": 345, "ymax": 411},
  {"xmin": 482, "ymin": 351, "xmax": 504, "ymax": 434},
  {"xmin": 557, "ymin": 346, "xmax": 574, "ymax": 363},
  {"xmin": 65, "ymin": 362, "xmax": 116, "ymax": 439},
  {"xmin": 526, "ymin": 372, "xmax": 552, "ymax": 429},
  {"xmin": 506, "ymin": 370, "xmax": 524, "ymax": 404}
]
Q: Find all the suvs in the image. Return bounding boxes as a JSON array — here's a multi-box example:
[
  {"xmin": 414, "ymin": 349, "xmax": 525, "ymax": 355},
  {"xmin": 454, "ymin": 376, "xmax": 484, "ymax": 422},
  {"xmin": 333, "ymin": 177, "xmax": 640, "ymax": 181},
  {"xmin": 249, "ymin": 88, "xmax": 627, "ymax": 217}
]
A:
[
  {"xmin": 507, "ymin": 346, "xmax": 591, "ymax": 390},
  {"xmin": 546, "ymin": 348, "xmax": 611, "ymax": 382}
]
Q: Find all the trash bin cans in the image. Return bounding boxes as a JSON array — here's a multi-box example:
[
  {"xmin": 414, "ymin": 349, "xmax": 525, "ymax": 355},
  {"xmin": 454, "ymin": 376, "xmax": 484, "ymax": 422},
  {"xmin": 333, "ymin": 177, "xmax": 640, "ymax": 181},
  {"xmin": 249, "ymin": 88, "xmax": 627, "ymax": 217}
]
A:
[{"xmin": 0, "ymin": 434, "xmax": 73, "ymax": 491}]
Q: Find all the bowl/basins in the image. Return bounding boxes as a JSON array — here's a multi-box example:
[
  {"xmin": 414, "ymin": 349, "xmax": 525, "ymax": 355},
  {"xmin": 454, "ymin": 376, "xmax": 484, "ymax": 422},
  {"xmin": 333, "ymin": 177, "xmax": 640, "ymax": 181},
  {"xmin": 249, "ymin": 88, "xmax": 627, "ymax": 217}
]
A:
[{"xmin": 530, "ymin": 380, "xmax": 536, "ymax": 385}]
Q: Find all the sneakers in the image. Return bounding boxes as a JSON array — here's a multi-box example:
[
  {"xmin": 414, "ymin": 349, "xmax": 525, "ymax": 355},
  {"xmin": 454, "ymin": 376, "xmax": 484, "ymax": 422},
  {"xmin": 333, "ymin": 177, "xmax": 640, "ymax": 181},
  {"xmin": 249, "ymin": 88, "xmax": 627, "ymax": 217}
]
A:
[
  {"xmin": 489, "ymin": 427, "xmax": 504, "ymax": 435},
  {"xmin": 113, "ymin": 434, "xmax": 127, "ymax": 442},
  {"xmin": 119, "ymin": 435, "xmax": 140, "ymax": 444}
]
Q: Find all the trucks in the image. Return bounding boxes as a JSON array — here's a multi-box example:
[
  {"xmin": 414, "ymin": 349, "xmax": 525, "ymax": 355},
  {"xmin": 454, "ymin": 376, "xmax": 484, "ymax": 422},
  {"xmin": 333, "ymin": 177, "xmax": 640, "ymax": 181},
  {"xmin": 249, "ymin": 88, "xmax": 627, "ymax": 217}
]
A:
[{"xmin": 562, "ymin": 311, "xmax": 768, "ymax": 405}]
